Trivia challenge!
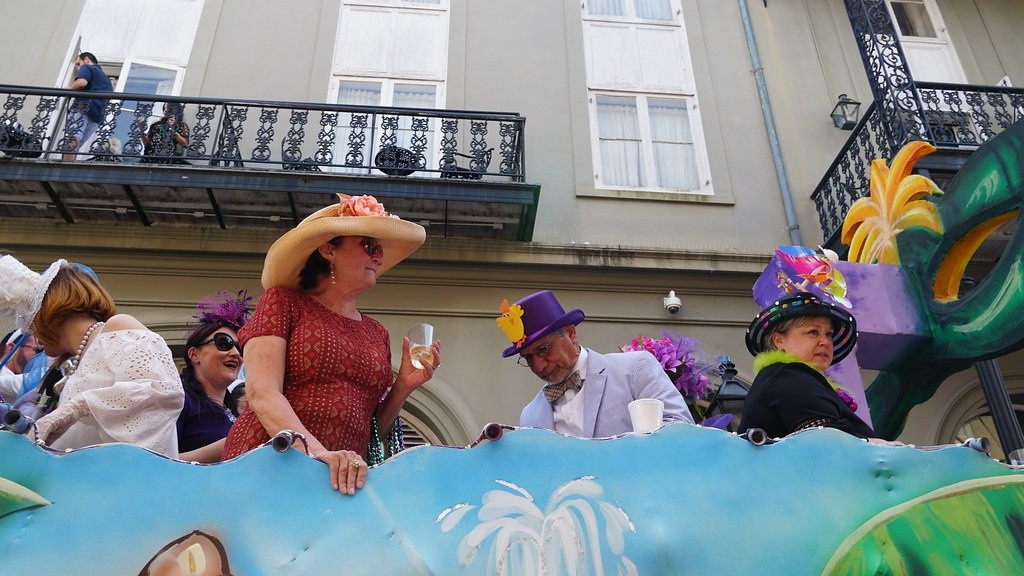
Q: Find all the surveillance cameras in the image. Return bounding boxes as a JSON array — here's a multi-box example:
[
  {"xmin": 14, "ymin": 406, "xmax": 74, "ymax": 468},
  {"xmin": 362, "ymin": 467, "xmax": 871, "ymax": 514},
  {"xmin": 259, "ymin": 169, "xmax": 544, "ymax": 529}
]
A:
[{"xmin": 666, "ymin": 296, "xmax": 681, "ymax": 314}]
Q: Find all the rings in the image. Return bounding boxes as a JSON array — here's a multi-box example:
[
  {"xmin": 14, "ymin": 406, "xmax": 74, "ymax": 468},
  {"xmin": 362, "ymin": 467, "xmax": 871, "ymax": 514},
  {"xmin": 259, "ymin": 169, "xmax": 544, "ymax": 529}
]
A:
[
  {"xmin": 433, "ymin": 362, "xmax": 441, "ymax": 369},
  {"xmin": 349, "ymin": 459, "xmax": 360, "ymax": 468}
]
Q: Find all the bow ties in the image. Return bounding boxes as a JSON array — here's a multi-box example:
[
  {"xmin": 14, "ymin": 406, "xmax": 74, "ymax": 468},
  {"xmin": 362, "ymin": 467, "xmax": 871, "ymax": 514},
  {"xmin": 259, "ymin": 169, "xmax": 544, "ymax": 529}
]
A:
[{"xmin": 542, "ymin": 370, "xmax": 582, "ymax": 403}]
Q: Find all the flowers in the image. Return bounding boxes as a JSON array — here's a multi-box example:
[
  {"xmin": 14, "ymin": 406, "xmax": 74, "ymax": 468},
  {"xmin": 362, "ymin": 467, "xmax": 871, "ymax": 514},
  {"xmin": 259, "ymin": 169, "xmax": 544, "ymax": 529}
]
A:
[
  {"xmin": 335, "ymin": 190, "xmax": 400, "ymax": 219},
  {"xmin": 619, "ymin": 330, "xmax": 718, "ymax": 419}
]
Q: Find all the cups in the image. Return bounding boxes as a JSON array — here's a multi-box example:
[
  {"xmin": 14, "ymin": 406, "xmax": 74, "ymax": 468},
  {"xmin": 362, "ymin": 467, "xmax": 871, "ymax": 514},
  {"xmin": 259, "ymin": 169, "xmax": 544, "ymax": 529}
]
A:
[
  {"xmin": 627, "ymin": 398, "xmax": 665, "ymax": 433},
  {"xmin": 1008, "ymin": 449, "xmax": 1024, "ymax": 470},
  {"xmin": 408, "ymin": 323, "xmax": 438, "ymax": 370}
]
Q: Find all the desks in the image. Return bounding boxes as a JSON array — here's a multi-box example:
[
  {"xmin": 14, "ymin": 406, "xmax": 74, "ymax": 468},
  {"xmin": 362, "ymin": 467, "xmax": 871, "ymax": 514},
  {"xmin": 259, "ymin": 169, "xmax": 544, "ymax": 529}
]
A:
[{"xmin": 375, "ymin": 146, "xmax": 416, "ymax": 178}]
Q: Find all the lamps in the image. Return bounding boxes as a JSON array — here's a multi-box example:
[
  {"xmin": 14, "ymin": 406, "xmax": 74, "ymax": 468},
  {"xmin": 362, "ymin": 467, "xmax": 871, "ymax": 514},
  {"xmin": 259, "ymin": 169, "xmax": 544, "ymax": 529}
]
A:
[{"xmin": 829, "ymin": 93, "xmax": 862, "ymax": 131}]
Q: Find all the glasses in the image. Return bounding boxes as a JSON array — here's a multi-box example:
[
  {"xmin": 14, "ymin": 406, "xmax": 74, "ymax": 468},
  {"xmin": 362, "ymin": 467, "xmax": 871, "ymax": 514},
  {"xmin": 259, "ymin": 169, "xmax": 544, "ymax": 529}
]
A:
[
  {"xmin": 329, "ymin": 237, "xmax": 384, "ymax": 259},
  {"xmin": 517, "ymin": 328, "xmax": 560, "ymax": 367},
  {"xmin": 15, "ymin": 344, "xmax": 44, "ymax": 354},
  {"xmin": 192, "ymin": 333, "xmax": 243, "ymax": 358}
]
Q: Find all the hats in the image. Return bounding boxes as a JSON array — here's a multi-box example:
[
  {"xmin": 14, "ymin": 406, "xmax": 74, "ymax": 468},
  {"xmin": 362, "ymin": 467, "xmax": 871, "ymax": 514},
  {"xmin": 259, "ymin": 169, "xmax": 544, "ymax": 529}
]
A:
[
  {"xmin": 261, "ymin": 192, "xmax": 426, "ymax": 291},
  {"xmin": 0, "ymin": 254, "xmax": 68, "ymax": 336},
  {"xmin": 496, "ymin": 289, "xmax": 585, "ymax": 358},
  {"xmin": 745, "ymin": 245, "xmax": 858, "ymax": 366}
]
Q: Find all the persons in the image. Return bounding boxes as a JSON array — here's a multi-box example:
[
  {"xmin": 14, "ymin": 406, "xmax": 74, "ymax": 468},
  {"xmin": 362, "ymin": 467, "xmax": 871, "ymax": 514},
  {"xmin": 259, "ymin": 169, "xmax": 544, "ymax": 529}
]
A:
[
  {"xmin": 174, "ymin": 289, "xmax": 252, "ymax": 464},
  {"xmin": 502, "ymin": 289, "xmax": 695, "ymax": 439},
  {"xmin": 64, "ymin": 52, "xmax": 113, "ymax": 161},
  {"xmin": 221, "ymin": 193, "xmax": 441, "ymax": 495},
  {"xmin": 738, "ymin": 245, "xmax": 875, "ymax": 439},
  {"xmin": 137, "ymin": 102, "xmax": 189, "ymax": 163},
  {"xmin": 0, "ymin": 254, "xmax": 185, "ymax": 460}
]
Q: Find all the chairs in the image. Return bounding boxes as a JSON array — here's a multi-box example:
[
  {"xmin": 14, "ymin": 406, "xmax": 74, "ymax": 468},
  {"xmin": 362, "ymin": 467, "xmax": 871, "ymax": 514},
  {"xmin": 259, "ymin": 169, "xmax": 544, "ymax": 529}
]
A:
[
  {"xmin": 440, "ymin": 147, "xmax": 495, "ymax": 180},
  {"xmin": 282, "ymin": 135, "xmax": 323, "ymax": 171}
]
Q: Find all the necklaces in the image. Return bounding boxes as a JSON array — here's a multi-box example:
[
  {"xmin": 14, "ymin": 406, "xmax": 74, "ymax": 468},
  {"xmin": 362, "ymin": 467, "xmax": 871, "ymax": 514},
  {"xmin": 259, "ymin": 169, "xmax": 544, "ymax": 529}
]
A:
[{"xmin": 70, "ymin": 321, "xmax": 105, "ymax": 374}]
